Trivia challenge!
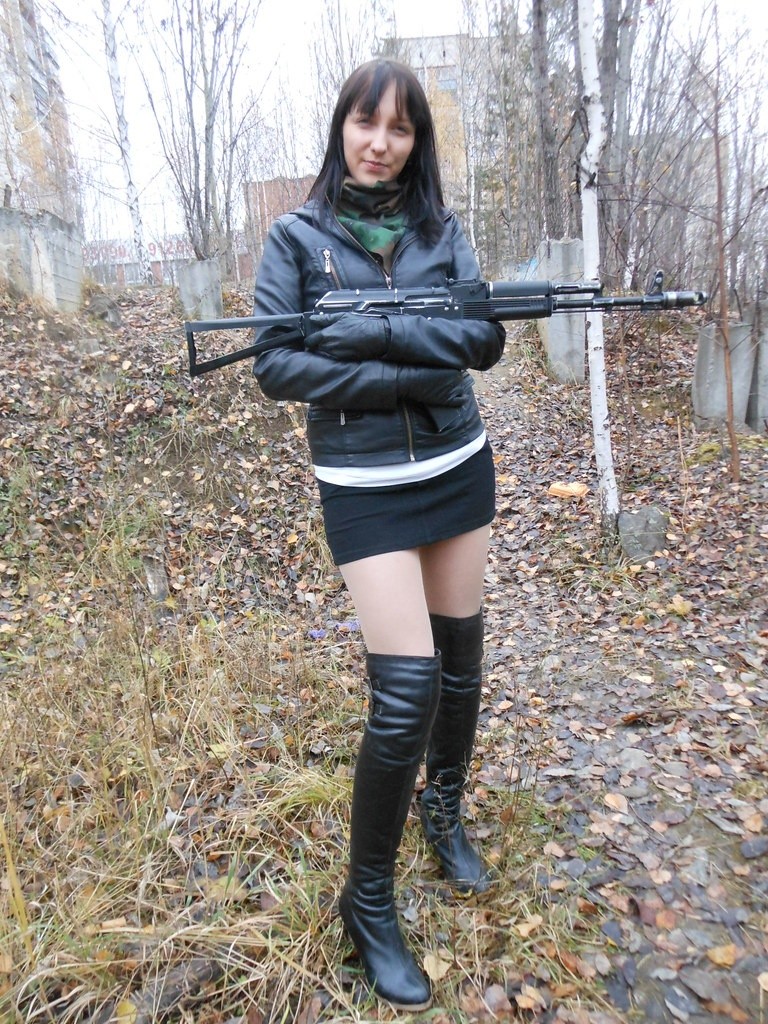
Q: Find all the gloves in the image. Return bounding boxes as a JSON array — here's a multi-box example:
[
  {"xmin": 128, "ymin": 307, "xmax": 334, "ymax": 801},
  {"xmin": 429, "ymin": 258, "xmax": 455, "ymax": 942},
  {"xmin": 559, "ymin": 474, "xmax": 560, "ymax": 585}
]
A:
[
  {"xmin": 303, "ymin": 315, "xmax": 387, "ymax": 364},
  {"xmin": 396, "ymin": 363, "xmax": 470, "ymax": 407}
]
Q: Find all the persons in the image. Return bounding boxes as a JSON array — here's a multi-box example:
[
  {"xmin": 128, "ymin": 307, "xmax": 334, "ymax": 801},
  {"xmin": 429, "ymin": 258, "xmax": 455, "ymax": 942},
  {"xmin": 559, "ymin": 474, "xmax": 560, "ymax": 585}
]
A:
[{"xmin": 254, "ymin": 57, "xmax": 507, "ymax": 1011}]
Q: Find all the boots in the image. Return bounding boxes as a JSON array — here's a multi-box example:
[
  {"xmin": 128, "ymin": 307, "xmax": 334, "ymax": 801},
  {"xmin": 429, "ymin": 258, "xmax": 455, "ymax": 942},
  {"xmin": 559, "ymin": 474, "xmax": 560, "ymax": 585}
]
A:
[
  {"xmin": 339, "ymin": 649, "xmax": 433, "ymax": 1011},
  {"xmin": 421, "ymin": 605, "xmax": 496, "ymax": 901}
]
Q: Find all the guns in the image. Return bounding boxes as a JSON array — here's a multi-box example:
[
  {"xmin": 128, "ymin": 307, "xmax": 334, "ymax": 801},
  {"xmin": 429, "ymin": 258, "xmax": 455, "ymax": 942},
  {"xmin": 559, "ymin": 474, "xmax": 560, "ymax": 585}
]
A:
[{"xmin": 182, "ymin": 266, "xmax": 710, "ymax": 379}]
